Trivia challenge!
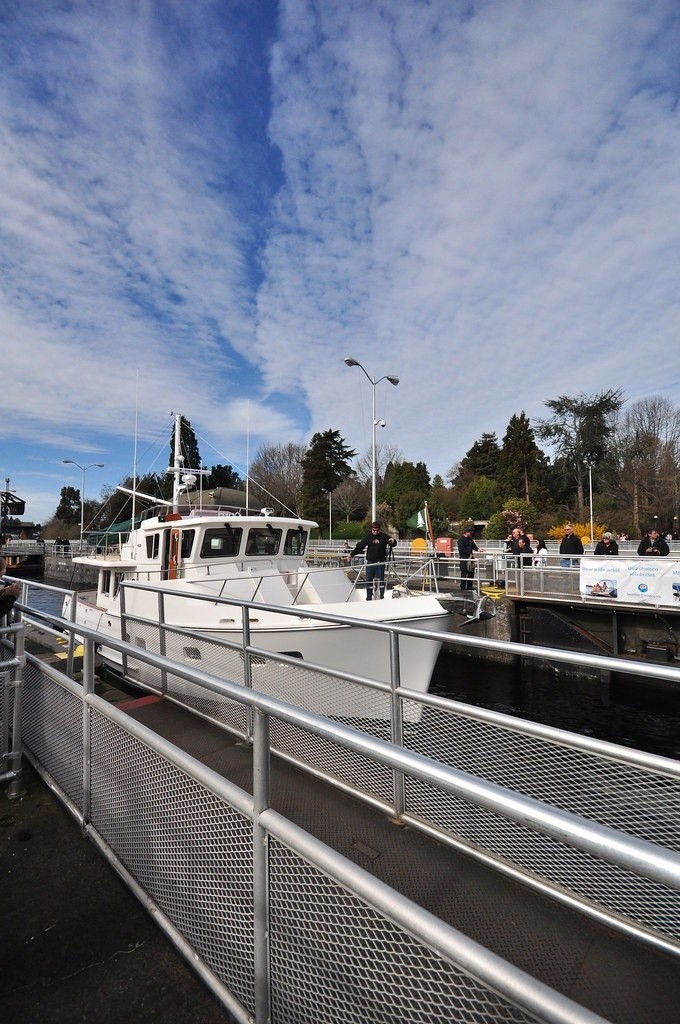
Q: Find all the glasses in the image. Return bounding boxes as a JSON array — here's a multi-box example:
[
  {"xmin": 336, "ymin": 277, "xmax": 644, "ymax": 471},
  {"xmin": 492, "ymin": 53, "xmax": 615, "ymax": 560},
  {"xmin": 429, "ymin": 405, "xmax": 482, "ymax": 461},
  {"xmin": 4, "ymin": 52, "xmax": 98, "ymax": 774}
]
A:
[{"xmin": 603, "ymin": 537, "xmax": 609, "ymax": 539}]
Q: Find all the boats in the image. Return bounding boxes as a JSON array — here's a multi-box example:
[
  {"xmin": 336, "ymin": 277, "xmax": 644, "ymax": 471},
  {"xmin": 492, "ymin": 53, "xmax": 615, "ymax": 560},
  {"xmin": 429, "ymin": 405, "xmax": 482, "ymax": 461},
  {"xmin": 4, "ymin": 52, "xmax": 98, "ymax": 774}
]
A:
[
  {"xmin": 0, "ymin": 476, "xmax": 46, "ymax": 585},
  {"xmin": 59, "ymin": 409, "xmax": 498, "ymax": 731}
]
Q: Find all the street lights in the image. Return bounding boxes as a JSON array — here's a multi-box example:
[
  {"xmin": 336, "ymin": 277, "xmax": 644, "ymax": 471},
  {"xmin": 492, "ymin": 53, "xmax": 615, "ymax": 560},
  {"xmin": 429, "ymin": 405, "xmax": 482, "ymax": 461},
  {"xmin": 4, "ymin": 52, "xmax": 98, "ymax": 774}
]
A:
[
  {"xmin": 343, "ymin": 356, "xmax": 402, "ymax": 526},
  {"xmin": 582, "ymin": 459, "xmax": 596, "ymax": 548},
  {"xmin": 322, "ymin": 488, "xmax": 332, "ymax": 545},
  {"xmin": 62, "ymin": 458, "xmax": 105, "ymax": 557}
]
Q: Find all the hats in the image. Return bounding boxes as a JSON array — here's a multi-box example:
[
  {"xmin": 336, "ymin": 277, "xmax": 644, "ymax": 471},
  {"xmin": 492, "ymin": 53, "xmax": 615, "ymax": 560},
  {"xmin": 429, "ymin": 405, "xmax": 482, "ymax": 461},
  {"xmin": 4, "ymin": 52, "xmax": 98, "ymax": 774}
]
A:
[
  {"xmin": 565, "ymin": 524, "xmax": 573, "ymax": 529},
  {"xmin": 465, "ymin": 528, "xmax": 472, "ymax": 531}
]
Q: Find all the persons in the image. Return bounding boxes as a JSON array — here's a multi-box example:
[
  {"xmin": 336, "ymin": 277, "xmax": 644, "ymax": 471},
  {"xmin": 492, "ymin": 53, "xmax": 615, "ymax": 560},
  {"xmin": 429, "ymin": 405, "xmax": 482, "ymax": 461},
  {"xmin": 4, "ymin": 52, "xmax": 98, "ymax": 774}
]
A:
[
  {"xmin": 594, "ymin": 532, "xmax": 619, "ymax": 555},
  {"xmin": 19, "ymin": 530, "xmax": 27, "ymax": 540},
  {"xmin": 348, "ymin": 522, "xmax": 397, "ymax": 601},
  {"xmin": 637, "ymin": 529, "xmax": 669, "ymax": 556},
  {"xmin": 54, "ymin": 536, "xmax": 70, "ymax": 554},
  {"xmin": 341, "ymin": 543, "xmax": 353, "ymax": 567},
  {"xmin": 0, "ymin": 584, "xmax": 21, "ymax": 619},
  {"xmin": 593, "ymin": 582, "xmax": 607, "ymax": 592},
  {"xmin": 35, "ymin": 531, "xmax": 45, "ymax": 546},
  {"xmin": 531, "ymin": 539, "xmax": 548, "ymax": 566},
  {"xmin": 662, "ymin": 530, "xmax": 679, "ymax": 540},
  {"xmin": 559, "ymin": 524, "xmax": 584, "ymax": 590},
  {"xmin": 619, "ymin": 534, "xmax": 630, "ymax": 540},
  {"xmin": 503, "ymin": 528, "xmax": 533, "ymax": 566},
  {"xmin": 351, "ymin": 542, "xmax": 364, "ymax": 569},
  {"xmin": 457, "ymin": 527, "xmax": 485, "ymax": 590}
]
